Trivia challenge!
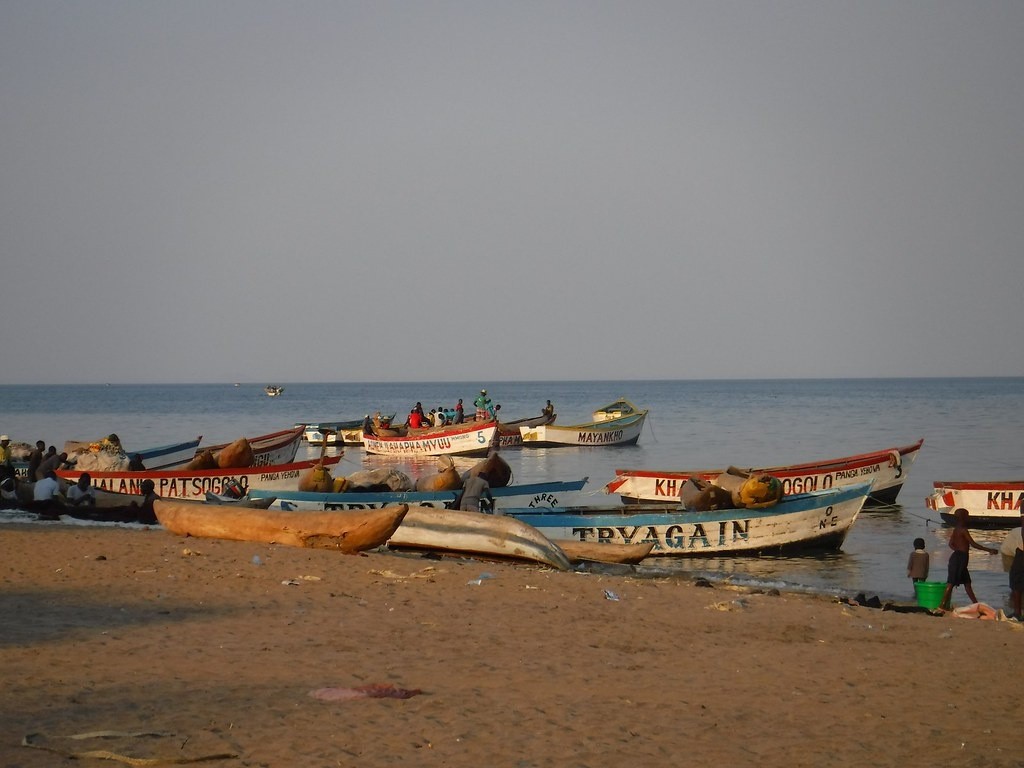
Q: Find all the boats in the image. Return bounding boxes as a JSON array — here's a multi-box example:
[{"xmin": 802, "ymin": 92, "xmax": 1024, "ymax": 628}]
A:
[
  {"xmin": 518, "ymin": 397, "xmax": 648, "ymax": 446},
  {"xmin": 548, "ymin": 539, "xmax": 662, "ymax": 564},
  {"xmin": 152, "ymin": 496, "xmax": 409, "ymax": 553},
  {"xmin": 193, "ymin": 421, "xmax": 308, "ymax": 470},
  {"xmin": 381, "ymin": 501, "xmax": 573, "ymax": 571},
  {"xmin": 340, "ymin": 423, "xmax": 411, "ymax": 446},
  {"xmin": 360, "ymin": 421, "xmax": 501, "ymax": 456},
  {"xmin": 14, "ymin": 473, "xmax": 279, "ymax": 520},
  {"xmin": 493, "ymin": 475, "xmax": 877, "ymax": 556},
  {"xmin": 9, "ymin": 433, "xmax": 205, "ymax": 479},
  {"xmin": 495, "ymin": 412, "xmax": 557, "ymax": 448},
  {"xmin": 382, "ymin": 502, "xmax": 575, "ymax": 574},
  {"xmin": 925, "ymin": 480, "xmax": 1024, "ymax": 526},
  {"xmin": 262, "ymin": 386, "xmax": 283, "ymax": 397},
  {"xmin": 52, "ymin": 451, "xmax": 346, "ymax": 497},
  {"xmin": 293, "ymin": 411, "xmax": 400, "ymax": 446},
  {"xmin": 205, "ymin": 474, "xmax": 597, "ymax": 514},
  {"xmin": 601, "ymin": 437, "xmax": 927, "ymax": 506}
]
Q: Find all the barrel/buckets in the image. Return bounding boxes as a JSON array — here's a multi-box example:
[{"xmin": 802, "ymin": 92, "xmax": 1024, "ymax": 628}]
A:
[{"xmin": 914, "ymin": 581, "xmax": 951, "ymax": 609}]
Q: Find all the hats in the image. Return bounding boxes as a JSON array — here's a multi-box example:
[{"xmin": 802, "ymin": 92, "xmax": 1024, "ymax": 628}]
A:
[
  {"xmin": 480, "ymin": 390, "xmax": 486, "ymax": 395},
  {"xmin": 0, "ymin": 435, "xmax": 12, "ymax": 441}
]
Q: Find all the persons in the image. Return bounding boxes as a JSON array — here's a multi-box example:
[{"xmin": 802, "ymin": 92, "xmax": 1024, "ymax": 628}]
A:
[
  {"xmin": 542, "ymin": 400, "xmax": 553, "ymax": 417},
  {"xmin": 66, "ymin": 473, "xmax": 105, "ymax": 520},
  {"xmin": 404, "ymin": 398, "xmax": 465, "ymax": 429},
  {"xmin": 131, "ymin": 478, "xmax": 161, "ymax": 524},
  {"xmin": 0, "ymin": 434, "xmax": 77, "ymax": 510},
  {"xmin": 937, "ymin": 501, "xmax": 1024, "ymax": 620},
  {"xmin": 362, "ymin": 414, "xmax": 379, "ymax": 439},
  {"xmin": 456, "ymin": 471, "xmax": 495, "ymax": 513},
  {"xmin": 907, "ymin": 537, "xmax": 930, "ymax": 584},
  {"xmin": 473, "ymin": 389, "xmax": 501, "ymax": 421},
  {"xmin": 32, "ymin": 471, "xmax": 67, "ymax": 520}
]
